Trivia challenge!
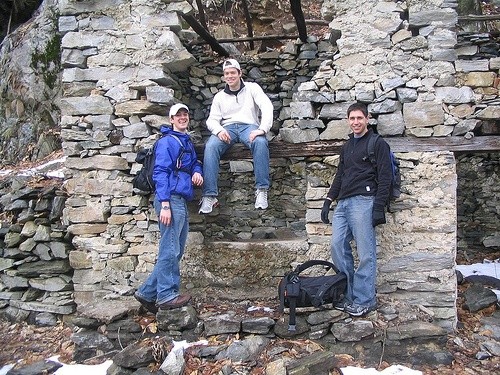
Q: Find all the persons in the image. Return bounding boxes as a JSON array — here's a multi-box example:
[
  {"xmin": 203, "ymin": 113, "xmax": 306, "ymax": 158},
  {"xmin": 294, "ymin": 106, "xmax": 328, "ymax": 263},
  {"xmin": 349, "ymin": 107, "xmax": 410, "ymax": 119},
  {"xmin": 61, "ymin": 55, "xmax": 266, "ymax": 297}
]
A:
[
  {"xmin": 134, "ymin": 103, "xmax": 204, "ymax": 314},
  {"xmin": 321, "ymin": 103, "xmax": 393, "ymax": 317},
  {"xmin": 198, "ymin": 59, "xmax": 274, "ymax": 216}
]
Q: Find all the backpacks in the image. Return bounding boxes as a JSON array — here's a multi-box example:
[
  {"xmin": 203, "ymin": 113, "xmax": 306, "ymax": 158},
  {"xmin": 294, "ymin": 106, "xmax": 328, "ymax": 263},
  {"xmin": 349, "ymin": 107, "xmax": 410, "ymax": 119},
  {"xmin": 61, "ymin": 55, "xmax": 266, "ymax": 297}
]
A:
[
  {"xmin": 367, "ymin": 134, "xmax": 401, "ymax": 201},
  {"xmin": 132, "ymin": 134, "xmax": 192, "ymax": 197},
  {"xmin": 278, "ymin": 260, "xmax": 348, "ymax": 331}
]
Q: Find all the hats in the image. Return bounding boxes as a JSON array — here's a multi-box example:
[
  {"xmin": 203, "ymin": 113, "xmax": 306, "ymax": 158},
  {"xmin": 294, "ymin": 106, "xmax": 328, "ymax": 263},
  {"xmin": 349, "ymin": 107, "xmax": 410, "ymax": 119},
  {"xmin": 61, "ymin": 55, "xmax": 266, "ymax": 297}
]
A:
[
  {"xmin": 223, "ymin": 59, "xmax": 240, "ymax": 71},
  {"xmin": 169, "ymin": 103, "xmax": 189, "ymax": 118}
]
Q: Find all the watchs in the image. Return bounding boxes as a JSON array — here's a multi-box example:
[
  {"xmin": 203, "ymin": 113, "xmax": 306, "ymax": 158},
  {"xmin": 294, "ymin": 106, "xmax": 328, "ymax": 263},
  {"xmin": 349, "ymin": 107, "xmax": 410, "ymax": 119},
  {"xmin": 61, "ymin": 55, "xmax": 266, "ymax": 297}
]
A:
[{"xmin": 161, "ymin": 205, "xmax": 170, "ymax": 210}]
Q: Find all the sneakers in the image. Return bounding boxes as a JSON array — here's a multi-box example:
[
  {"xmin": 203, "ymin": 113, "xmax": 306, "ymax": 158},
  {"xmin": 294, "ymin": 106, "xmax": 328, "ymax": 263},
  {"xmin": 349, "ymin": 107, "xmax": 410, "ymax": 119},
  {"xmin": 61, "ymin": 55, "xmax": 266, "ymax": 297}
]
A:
[
  {"xmin": 198, "ymin": 196, "xmax": 219, "ymax": 214},
  {"xmin": 160, "ymin": 295, "xmax": 191, "ymax": 310},
  {"xmin": 345, "ymin": 300, "xmax": 378, "ymax": 316},
  {"xmin": 254, "ymin": 189, "xmax": 269, "ymax": 209},
  {"xmin": 134, "ymin": 294, "xmax": 158, "ymax": 314},
  {"xmin": 334, "ymin": 297, "xmax": 351, "ymax": 310}
]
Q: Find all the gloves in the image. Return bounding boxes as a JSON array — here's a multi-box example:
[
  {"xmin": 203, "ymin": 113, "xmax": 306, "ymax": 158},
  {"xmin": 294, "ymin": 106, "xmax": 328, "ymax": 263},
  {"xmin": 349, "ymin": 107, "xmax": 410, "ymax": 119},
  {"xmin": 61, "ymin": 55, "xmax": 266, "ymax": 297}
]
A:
[
  {"xmin": 321, "ymin": 199, "xmax": 332, "ymax": 224},
  {"xmin": 372, "ymin": 204, "xmax": 386, "ymax": 227}
]
